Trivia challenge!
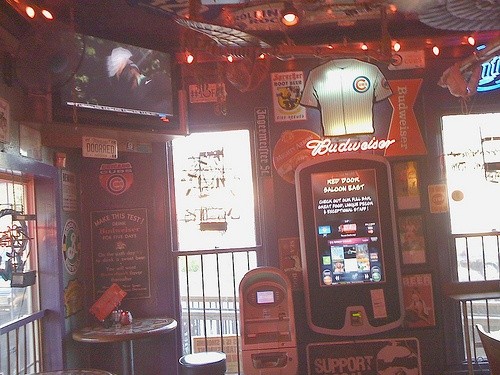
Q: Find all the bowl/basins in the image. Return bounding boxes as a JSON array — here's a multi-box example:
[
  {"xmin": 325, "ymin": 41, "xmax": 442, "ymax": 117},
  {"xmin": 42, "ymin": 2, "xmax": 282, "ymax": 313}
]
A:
[{"xmin": 99, "ymin": 318, "xmax": 115, "ymax": 329}]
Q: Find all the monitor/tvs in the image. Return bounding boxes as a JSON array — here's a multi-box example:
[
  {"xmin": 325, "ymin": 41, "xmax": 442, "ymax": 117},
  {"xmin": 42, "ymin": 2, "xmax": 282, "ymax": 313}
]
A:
[
  {"xmin": 309, "ymin": 167, "xmax": 385, "ymax": 288},
  {"xmin": 50, "ymin": 27, "xmax": 180, "ymax": 131}
]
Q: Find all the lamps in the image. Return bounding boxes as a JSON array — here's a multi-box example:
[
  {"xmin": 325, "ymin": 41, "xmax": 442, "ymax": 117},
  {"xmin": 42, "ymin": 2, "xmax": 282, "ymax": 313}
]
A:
[
  {"xmin": 280, "ymin": 0, "xmax": 301, "ymax": 27},
  {"xmin": 328, "ymin": 40, "xmax": 450, "ymax": 56},
  {"xmin": 174, "ymin": 49, "xmax": 265, "ymax": 63}
]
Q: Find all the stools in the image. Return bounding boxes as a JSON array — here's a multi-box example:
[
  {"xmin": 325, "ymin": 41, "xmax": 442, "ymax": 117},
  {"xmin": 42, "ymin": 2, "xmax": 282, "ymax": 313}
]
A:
[{"xmin": 178, "ymin": 351, "xmax": 227, "ymax": 375}]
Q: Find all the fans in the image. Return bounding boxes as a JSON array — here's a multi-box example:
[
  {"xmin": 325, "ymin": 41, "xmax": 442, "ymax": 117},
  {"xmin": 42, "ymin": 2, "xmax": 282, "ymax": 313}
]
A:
[{"xmin": 0, "ymin": 21, "xmax": 86, "ymax": 95}]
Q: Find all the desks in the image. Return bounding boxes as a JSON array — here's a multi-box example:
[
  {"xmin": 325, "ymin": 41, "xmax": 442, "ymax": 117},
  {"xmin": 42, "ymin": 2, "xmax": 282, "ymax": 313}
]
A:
[{"xmin": 72, "ymin": 317, "xmax": 177, "ymax": 375}]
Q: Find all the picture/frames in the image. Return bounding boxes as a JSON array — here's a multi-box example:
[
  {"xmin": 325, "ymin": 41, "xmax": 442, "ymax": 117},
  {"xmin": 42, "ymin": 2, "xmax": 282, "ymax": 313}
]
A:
[
  {"xmin": 390, "ymin": 158, "xmax": 424, "ymax": 212},
  {"xmin": 397, "ymin": 213, "xmax": 431, "ymax": 267},
  {"xmin": 394, "ymin": 271, "xmax": 438, "ymax": 332}
]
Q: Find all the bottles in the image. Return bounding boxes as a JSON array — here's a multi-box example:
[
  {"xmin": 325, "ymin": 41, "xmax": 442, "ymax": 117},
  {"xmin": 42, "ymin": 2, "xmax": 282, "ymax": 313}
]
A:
[
  {"xmin": 112, "ymin": 310, "xmax": 118, "ymax": 324},
  {"xmin": 120, "ymin": 311, "xmax": 133, "ymax": 326},
  {"xmin": 118, "ymin": 309, "xmax": 123, "ymax": 322}
]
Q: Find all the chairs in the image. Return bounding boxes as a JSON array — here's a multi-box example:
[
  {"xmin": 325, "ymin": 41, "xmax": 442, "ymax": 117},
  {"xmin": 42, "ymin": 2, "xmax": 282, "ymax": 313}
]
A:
[{"xmin": 476, "ymin": 324, "xmax": 500, "ymax": 375}]
